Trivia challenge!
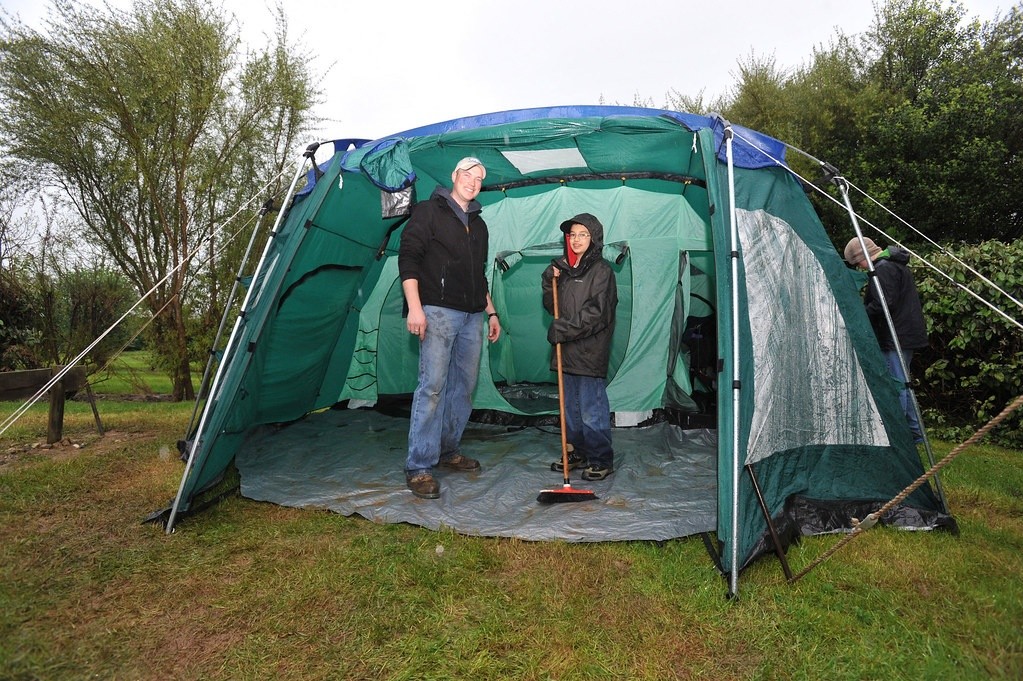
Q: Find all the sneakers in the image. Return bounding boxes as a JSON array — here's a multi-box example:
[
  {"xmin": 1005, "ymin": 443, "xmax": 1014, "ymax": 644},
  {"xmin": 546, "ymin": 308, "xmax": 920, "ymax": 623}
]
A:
[
  {"xmin": 551, "ymin": 451, "xmax": 591, "ymax": 471},
  {"xmin": 581, "ymin": 464, "xmax": 613, "ymax": 480}
]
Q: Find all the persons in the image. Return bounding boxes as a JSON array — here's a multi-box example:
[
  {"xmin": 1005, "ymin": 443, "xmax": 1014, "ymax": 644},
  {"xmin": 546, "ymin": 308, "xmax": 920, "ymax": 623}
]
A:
[
  {"xmin": 540, "ymin": 213, "xmax": 620, "ymax": 481},
  {"xmin": 844, "ymin": 237, "xmax": 926, "ymax": 444},
  {"xmin": 397, "ymin": 157, "xmax": 502, "ymax": 499}
]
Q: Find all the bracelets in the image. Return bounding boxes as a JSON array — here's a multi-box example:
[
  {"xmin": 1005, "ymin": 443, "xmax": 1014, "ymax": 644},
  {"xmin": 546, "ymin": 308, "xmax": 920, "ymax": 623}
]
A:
[{"xmin": 487, "ymin": 313, "xmax": 500, "ymax": 320}]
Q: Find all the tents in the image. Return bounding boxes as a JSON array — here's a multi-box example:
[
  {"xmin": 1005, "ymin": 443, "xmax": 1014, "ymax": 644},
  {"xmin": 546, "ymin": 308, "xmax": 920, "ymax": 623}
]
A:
[{"xmin": 141, "ymin": 103, "xmax": 962, "ymax": 606}]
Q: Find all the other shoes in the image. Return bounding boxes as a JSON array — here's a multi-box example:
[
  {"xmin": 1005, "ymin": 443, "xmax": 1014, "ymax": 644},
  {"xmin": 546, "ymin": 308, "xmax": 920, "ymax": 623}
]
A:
[
  {"xmin": 405, "ymin": 472, "xmax": 440, "ymax": 499},
  {"xmin": 433, "ymin": 453, "xmax": 482, "ymax": 472}
]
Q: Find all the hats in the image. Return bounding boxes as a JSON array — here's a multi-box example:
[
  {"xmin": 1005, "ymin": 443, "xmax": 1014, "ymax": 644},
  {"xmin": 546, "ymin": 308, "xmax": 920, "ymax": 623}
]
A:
[
  {"xmin": 844, "ymin": 236, "xmax": 882, "ymax": 264},
  {"xmin": 454, "ymin": 156, "xmax": 487, "ymax": 179}
]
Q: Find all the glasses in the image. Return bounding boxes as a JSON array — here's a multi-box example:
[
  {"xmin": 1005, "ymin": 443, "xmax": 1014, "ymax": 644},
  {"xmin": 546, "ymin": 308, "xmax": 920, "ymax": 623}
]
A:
[{"xmin": 569, "ymin": 230, "xmax": 591, "ymax": 239}]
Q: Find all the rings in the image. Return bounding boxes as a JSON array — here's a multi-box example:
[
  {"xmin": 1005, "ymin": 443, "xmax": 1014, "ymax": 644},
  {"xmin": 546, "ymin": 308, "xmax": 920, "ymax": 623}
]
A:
[{"xmin": 414, "ymin": 330, "xmax": 419, "ymax": 332}]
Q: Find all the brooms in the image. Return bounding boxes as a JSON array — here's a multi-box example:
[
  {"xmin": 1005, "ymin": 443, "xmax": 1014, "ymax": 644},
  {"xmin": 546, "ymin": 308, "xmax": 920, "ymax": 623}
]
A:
[{"xmin": 535, "ymin": 276, "xmax": 601, "ymax": 504}]
